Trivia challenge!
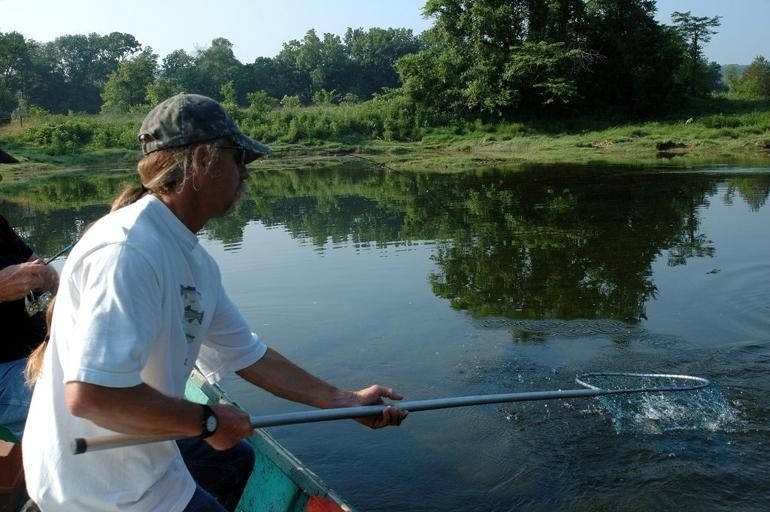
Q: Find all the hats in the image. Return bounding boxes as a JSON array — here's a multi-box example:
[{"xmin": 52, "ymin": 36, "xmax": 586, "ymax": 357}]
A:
[
  {"xmin": 0, "ymin": 149, "xmax": 19, "ymax": 163},
  {"xmin": 137, "ymin": 91, "xmax": 273, "ymax": 166}
]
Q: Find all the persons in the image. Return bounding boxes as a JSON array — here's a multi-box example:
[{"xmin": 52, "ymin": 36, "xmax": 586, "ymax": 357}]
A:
[
  {"xmin": 0, "ymin": 151, "xmax": 60, "ymax": 446},
  {"xmin": 22, "ymin": 92, "xmax": 408, "ymax": 510}
]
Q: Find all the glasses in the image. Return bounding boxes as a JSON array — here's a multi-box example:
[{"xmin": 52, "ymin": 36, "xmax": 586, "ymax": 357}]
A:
[{"xmin": 215, "ymin": 143, "xmax": 248, "ymax": 166}]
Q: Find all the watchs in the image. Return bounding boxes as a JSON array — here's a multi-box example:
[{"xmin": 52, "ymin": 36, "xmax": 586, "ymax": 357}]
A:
[{"xmin": 195, "ymin": 404, "xmax": 219, "ymax": 441}]
